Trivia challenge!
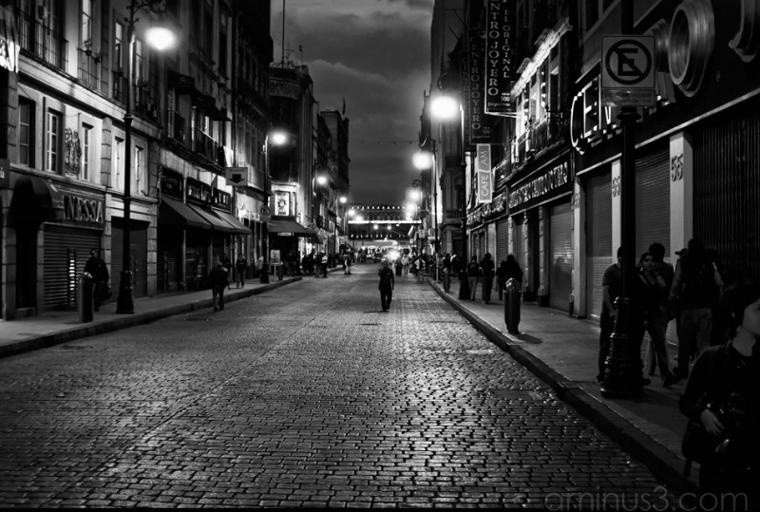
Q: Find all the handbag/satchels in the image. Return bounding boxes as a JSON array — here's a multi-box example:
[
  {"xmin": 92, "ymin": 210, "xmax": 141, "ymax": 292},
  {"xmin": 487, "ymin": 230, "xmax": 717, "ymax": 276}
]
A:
[{"xmin": 682, "ymin": 405, "xmax": 717, "ymax": 477}]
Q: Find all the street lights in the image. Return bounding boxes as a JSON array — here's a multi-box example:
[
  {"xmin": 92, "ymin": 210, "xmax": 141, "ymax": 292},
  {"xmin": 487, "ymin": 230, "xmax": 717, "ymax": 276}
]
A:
[
  {"xmin": 408, "ymin": 173, "xmax": 430, "ymax": 274},
  {"xmin": 310, "ymin": 164, "xmax": 327, "ymax": 268},
  {"xmin": 260, "ymin": 118, "xmax": 290, "ymax": 285},
  {"xmin": 114, "ymin": 1, "xmax": 180, "ymax": 315},
  {"xmin": 414, "ymin": 136, "xmax": 441, "ymax": 283},
  {"xmin": 334, "ymin": 187, "xmax": 425, "ymax": 269},
  {"xmin": 431, "ymin": 73, "xmax": 472, "ymax": 302}
]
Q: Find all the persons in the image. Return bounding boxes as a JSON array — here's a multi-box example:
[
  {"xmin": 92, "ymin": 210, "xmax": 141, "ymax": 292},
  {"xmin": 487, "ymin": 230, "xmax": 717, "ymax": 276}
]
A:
[
  {"xmin": 378, "ymin": 259, "xmax": 395, "ymax": 312},
  {"xmin": 209, "ymin": 242, "xmax": 523, "ymax": 304},
  {"xmin": 595, "ymin": 235, "xmax": 727, "ymax": 384},
  {"xmin": 209, "ymin": 261, "xmax": 228, "ymax": 312},
  {"xmin": 94, "ymin": 248, "xmax": 111, "ymax": 311},
  {"xmin": 679, "ymin": 280, "xmax": 758, "ymax": 512}
]
